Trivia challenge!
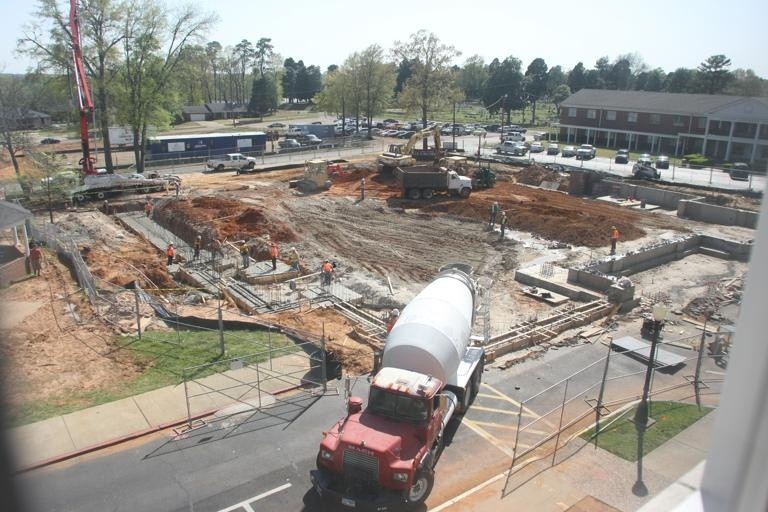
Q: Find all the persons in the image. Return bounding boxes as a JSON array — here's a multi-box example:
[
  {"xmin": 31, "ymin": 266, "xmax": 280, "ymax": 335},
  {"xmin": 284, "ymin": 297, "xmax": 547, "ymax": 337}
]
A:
[
  {"xmin": 166, "ymin": 241, "xmax": 175, "ymax": 266},
  {"xmin": 323, "ymin": 259, "xmax": 335, "ymax": 286},
  {"xmin": 487, "ymin": 201, "xmax": 499, "ymax": 228},
  {"xmin": 288, "ymin": 247, "xmax": 300, "ymax": 270},
  {"xmin": 194, "ymin": 235, "xmax": 202, "ymax": 260},
  {"xmin": 610, "ymin": 225, "xmax": 618, "ymax": 252},
  {"xmin": 359, "ymin": 178, "xmax": 365, "ymax": 200},
  {"xmin": 173, "ymin": 181, "xmax": 179, "ymax": 196},
  {"xmin": 499, "ymin": 211, "xmax": 507, "ymax": 235},
  {"xmin": 209, "ymin": 238, "xmax": 225, "ymax": 259},
  {"xmin": 239, "ymin": 240, "xmax": 250, "ymax": 269},
  {"xmin": 28, "ymin": 242, "xmax": 43, "ymax": 276},
  {"xmin": 145, "ymin": 201, "xmax": 153, "ymax": 217},
  {"xmin": 271, "ymin": 242, "xmax": 278, "ymax": 270}
]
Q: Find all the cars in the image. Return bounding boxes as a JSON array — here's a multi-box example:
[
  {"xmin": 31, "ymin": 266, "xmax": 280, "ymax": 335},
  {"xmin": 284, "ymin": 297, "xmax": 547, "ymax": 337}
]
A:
[
  {"xmin": 410, "ymin": 116, "xmax": 598, "ymax": 160},
  {"xmin": 638, "ymin": 153, "xmax": 652, "ymax": 166},
  {"xmin": 265, "ymin": 116, "xmax": 416, "ymax": 148},
  {"xmin": 40, "ymin": 137, "xmax": 62, "ymax": 145},
  {"xmin": 654, "ymin": 155, "xmax": 670, "ymax": 169}
]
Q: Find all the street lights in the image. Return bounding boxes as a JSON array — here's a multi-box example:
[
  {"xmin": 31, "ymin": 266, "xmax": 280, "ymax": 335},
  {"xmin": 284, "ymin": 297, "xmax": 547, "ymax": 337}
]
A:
[
  {"xmin": 631, "ymin": 300, "xmax": 669, "ymax": 424},
  {"xmin": 693, "ymin": 304, "xmax": 716, "ymax": 382}
]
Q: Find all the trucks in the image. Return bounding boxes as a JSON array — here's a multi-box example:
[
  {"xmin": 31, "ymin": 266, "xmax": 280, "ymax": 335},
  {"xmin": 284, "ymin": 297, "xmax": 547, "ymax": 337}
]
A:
[{"xmin": 392, "ymin": 164, "xmax": 473, "ymax": 199}]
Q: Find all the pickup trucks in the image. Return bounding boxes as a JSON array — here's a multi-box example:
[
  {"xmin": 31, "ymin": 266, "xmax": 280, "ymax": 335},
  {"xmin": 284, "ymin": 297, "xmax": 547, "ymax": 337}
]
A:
[{"xmin": 206, "ymin": 152, "xmax": 257, "ymax": 172}]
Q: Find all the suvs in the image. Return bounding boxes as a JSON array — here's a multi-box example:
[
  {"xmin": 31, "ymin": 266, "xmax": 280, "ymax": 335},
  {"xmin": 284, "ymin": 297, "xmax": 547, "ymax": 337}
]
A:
[{"xmin": 614, "ymin": 148, "xmax": 630, "ymax": 164}]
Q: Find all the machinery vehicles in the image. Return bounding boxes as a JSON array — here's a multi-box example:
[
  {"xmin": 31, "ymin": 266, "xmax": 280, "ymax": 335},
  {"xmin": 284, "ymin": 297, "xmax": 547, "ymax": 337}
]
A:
[
  {"xmin": 37, "ymin": 1, "xmax": 181, "ymax": 204},
  {"xmin": 288, "ymin": 157, "xmax": 346, "ymax": 193},
  {"xmin": 309, "ymin": 259, "xmax": 494, "ymax": 512},
  {"xmin": 377, "ymin": 118, "xmax": 443, "ymax": 178}
]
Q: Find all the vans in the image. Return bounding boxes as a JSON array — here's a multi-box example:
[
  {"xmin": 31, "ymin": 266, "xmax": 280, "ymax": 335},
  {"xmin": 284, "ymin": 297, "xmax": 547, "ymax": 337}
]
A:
[{"xmin": 730, "ymin": 161, "xmax": 749, "ymax": 180}]
